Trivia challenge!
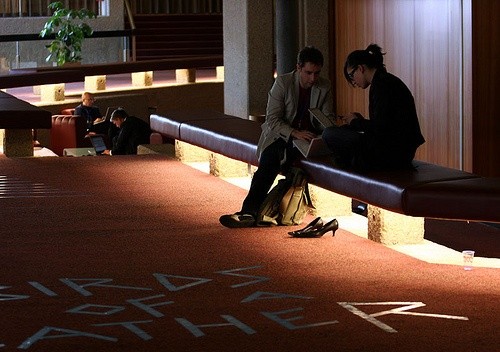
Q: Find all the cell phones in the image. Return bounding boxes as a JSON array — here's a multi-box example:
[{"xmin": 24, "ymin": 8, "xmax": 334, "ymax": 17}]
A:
[{"xmin": 334, "ymin": 112, "xmax": 347, "ymax": 120}]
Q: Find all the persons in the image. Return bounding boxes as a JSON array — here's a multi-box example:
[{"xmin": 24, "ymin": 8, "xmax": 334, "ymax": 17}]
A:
[
  {"xmin": 219, "ymin": 46, "xmax": 337, "ymax": 230},
  {"xmin": 318, "ymin": 41, "xmax": 425, "ymax": 174},
  {"xmin": 99, "ymin": 107, "xmax": 150, "ymax": 156},
  {"xmin": 73, "ymin": 92, "xmax": 105, "ymax": 140}
]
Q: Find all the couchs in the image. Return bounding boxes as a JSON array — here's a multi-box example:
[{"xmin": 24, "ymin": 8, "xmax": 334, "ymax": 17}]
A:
[
  {"xmin": 49, "ymin": 108, "xmax": 82, "ymax": 157},
  {"xmin": 150, "ymin": 132, "xmax": 174, "ymax": 144}
]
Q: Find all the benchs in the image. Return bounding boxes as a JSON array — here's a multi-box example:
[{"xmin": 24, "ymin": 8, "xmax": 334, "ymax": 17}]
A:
[
  {"xmin": 149, "ymin": 108, "xmax": 481, "ymax": 244},
  {"xmin": 403, "ymin": 174, "xmax": 500, "ymax": 223},
  {"xmin": 0, "ymin": 91, "xmax": 52, "ymax": 157}
]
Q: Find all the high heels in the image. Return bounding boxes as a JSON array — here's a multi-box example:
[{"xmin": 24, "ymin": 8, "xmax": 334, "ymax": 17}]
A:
[
  {"xmin": 288, "ymin": 217, "xmax": 323, "ymax": 236},
  {"xmin": 300, "ymin": 219, "xmax": 338, "ymax": 238}
]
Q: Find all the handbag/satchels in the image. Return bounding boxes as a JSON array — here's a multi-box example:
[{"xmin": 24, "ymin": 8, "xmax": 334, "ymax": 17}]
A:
[{"xmin": 256, "ymin": 179, "xmax": 316, "ymax": 227}]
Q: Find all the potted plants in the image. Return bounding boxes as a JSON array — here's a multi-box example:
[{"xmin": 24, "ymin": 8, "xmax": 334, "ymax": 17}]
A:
[{"xmin": 38, "ymin": 2, "xmax": 98, "ymax": 67}]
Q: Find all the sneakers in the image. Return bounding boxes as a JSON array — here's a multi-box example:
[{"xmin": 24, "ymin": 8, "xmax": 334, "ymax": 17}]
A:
[{"xmin": 219, "ymin": 212, "xmax": 256, "ymax": 228}]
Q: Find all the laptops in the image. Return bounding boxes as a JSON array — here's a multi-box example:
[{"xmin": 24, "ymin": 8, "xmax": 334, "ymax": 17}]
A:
[
  {"xmin": 306, "ymin": 105, "xmax": 358, "ymax": 132},
  {"xmin": 91, "ymin": 105, "xmax": 117, "ymax": 129},
  {"xmin": 292, "ymin": 136, "xmax": 322, "ymax": 158},
  {"xmin": 90, "ymin": 134, "xmax": 111, "ymax": 157}
]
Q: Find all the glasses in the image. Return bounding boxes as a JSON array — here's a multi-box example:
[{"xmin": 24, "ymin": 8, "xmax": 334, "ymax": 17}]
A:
[
  {"xmin": 84, "ymin": 97, "xmax": 96, "ymax": 102},
  {"xmin": 348, "ymin": 66, "xmax": 358, "ymax": 81}
]
